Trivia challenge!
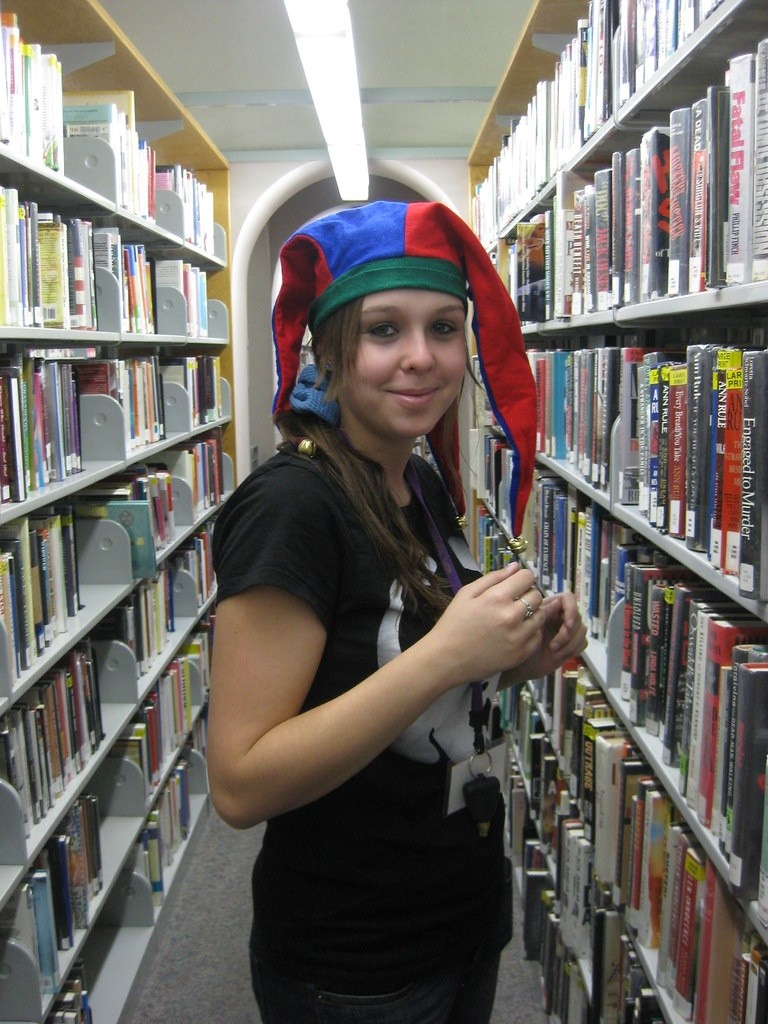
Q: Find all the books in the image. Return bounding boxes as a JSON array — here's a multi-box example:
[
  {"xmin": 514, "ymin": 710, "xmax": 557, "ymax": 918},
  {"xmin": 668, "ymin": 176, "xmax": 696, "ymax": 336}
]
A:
[
  {"xmin": 1, "ymin": 9, "xmax": 217, "ymax": 258},
  {"xmin": 0, "ymin": 614, "xmax": 215, "ymax": 1024},
  {"xmin": 0, "ymin": 347, "xmax": 223, "ymax": 506},
  {"xmin": 475, "ymin": 434, "xmax": 768, "ymax": 1024},
  {"xmin": 1, "ymin": 432, "xmax": 223, "ymax": 701},
  {"xmin": 0, "ymin": 185, "xmax": 209, "ymax": 340},
  {"xmin": 471, "ymin": 0, "xmax": 725, "ymax": 248},
  {"xmin": 0, "ymin": 519, "xmax": 216, "ymax": 839},
  {"xmin": 472, "ymin": 325, "xmax": 768, "ymax": 604},
  {"xmin": 489, "ymin": 38, "xmax": 768, "ymax": 327}
]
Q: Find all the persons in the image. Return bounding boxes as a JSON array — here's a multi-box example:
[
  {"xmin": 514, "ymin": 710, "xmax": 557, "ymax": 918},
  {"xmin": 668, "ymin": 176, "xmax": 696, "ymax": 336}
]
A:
[{"xmin": 208, "ymin": 200, "xmax": 589, "ymax": 1024}]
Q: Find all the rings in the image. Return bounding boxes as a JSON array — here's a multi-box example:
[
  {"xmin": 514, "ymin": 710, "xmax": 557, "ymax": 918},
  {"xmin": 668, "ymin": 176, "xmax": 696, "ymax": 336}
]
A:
[{"xmin": 516, "ymin": 597, "xmax": 533, "ymax": 620}]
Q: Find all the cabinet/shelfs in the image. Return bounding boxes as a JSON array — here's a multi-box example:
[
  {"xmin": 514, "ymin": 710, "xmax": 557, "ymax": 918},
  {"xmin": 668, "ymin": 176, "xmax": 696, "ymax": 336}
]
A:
[
  {"xmin": 0, "ymin": 0, "xmax": 234, "ymax": 1024},
  {"xmin": 466, "ymin": 0, "xmax": 768, "ymax": 1024}
]
